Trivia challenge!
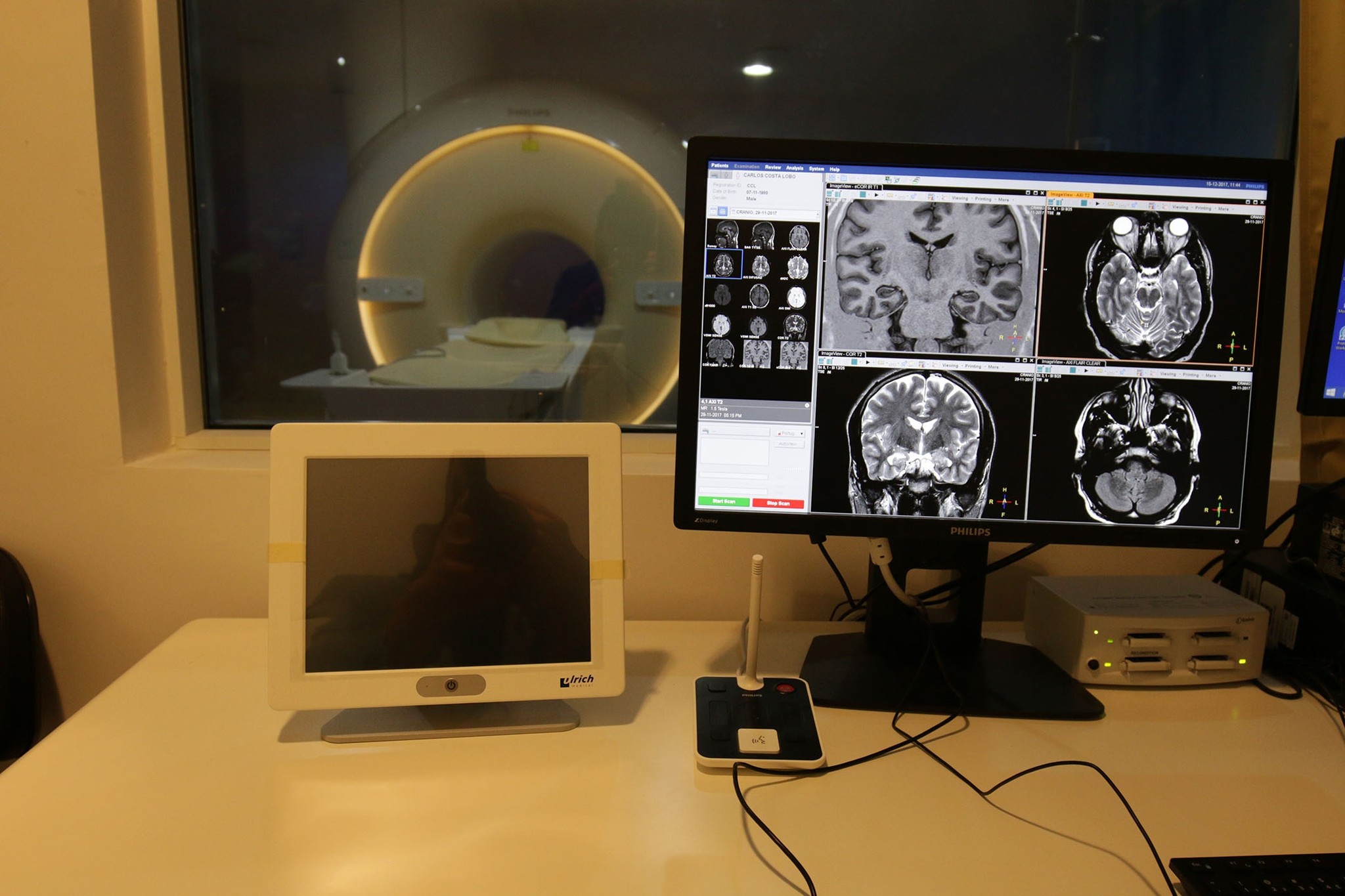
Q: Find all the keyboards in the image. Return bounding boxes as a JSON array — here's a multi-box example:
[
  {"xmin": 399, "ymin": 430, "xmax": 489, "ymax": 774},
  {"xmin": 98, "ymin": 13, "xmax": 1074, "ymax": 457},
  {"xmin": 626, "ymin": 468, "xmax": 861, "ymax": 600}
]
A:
[{"xmin": 1169, "ymin": 853, "xmax": 1345, "ymax": 896}]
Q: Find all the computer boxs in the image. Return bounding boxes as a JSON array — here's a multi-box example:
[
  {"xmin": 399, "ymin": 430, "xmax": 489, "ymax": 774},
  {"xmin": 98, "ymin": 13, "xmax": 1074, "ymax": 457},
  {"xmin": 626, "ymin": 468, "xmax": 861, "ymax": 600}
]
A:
[{"xmin": 1213, "ymin": 480, "xmax": 1344, "ymax": 712}]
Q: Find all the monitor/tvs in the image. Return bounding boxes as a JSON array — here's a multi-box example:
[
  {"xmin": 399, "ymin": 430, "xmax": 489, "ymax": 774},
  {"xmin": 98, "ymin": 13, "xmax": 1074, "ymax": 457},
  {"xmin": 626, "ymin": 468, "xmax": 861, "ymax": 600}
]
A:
[
  {"xmin": 671, "ymin": 139, "xmax": 1295, "ymax": 724},
  {"xmin": 1294, "ymin": 135, "xmax": 1345, "ymax": 417},
  {"xmin": 268, "ymin": 422, "xmax": 625, "ymax": 741}
]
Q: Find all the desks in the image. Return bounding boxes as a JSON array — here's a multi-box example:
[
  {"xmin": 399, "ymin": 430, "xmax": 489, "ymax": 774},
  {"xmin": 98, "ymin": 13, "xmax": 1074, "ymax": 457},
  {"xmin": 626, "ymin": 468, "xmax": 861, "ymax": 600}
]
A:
[{"xmin": 0, "ymin": 621, "xmax": 1345, "ymax": 896}]
